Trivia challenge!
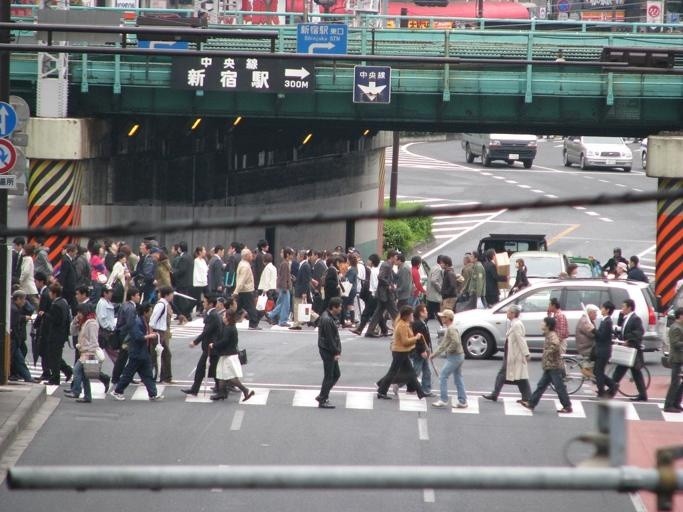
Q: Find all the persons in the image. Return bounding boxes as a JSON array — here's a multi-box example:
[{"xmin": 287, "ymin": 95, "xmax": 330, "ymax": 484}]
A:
[{"xmin": 8, "ymin": 236, "xmax": 683, "ymax": 414}]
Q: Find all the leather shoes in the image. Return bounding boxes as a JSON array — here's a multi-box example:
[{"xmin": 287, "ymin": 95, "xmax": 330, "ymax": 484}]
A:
[
  {"xmin": 316, "ymin": 396, "xmax": 336, "ymax": 408},
  {"xmin": 64, "ymin": 369, "xmax": 91, "ymax": 402},
  {"xmin": 104, "ymin": 376, "xmax": 110, "ymax": 393},
  {"xmin": 482, "ymin": 395, "xmax": 497, "ymax": 401},
  {"xmin": 181, "ymin": 388, "xmax": 197, "ymax": 396},
  {"xmin": 33, "ymin": 377, "xmax": 60, "ymax": 385}
]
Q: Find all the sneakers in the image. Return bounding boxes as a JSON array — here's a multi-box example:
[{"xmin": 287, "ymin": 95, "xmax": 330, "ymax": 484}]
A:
[
  {"xmin": 110, "ymin": 390, "xmax": 125, "ymax": 400},
  {"xmin": 453, "ymin": 402, "xmax": 467, "ymax": 408},
  {"xmin": 558, "ymin": 408, "xmax": 572, "ymax": 413},
  {"xmin": 150, "ymin": 395, "xmax": 164, "ymax": 400},
  {"xmin": 432, "ymin": 400, "xmax": 449, "ymax": 407},
  {"xmin": 521, "ymin": 401, "xmax": 534, "ymax": 409}
]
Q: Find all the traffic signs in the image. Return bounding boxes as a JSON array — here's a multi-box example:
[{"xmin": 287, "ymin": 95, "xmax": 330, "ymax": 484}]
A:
[
  {"xmin": 352, "ymin": 65, "xmax": 394, "ymax": 105},
  {"xmin": 169, "ymin": 53, "xmax": 316, "ymax": 95},
  {"xmin": 295, "ymin": 21, "xmax": 348, "ymax": 58}
]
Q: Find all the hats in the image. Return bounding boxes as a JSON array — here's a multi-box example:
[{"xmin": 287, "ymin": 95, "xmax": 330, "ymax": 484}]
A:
[
  {"xmin": 437, "ymin": 309, "xmax": 454, "ymax": 320},
  {"xmin": 617, "ymin": 262, "xmax": 628, "ymax": 271}
]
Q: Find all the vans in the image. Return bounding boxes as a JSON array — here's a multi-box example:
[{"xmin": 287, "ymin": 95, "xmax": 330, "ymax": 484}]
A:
[{"xmin": 434, "ymin": 277, "xmax": 664, "ymax": 356}]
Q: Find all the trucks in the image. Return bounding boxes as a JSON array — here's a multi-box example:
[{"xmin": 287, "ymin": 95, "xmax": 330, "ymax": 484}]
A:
[{"xmin": 459, "ymin": 131, "xmax": 541, "ymax": 169}]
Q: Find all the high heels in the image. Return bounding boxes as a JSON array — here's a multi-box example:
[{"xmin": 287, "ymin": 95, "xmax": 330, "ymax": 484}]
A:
[
  {"xmin": 377, "ymin": 393, "xmax": 392, "ymax": 399},
  {"xmin": 417, "ymin": 392, "xmax": 432, "ymax": 398}
]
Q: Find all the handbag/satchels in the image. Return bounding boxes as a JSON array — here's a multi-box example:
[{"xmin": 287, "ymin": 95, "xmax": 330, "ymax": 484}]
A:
[
  {"xmin": 298, "ymin": 303, "xmax": 312, "ymax": 322},
  {"xmin": 109, "ymin": 332, "xmax": 120, "ymax": 350},
  {"xmin": 82, "ymin": 359, "xmax": 102, "ymax": 379},
  {"xmin": 239, "ymin": 349, "xmax": 246, "ymax": 365},
  {"xmin": 609, "ymin": 344, "xmax": 638, "ymax": 367}
]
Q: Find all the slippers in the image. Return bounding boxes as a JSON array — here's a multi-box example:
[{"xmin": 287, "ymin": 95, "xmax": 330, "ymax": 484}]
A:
[
  {"xmin": 243, "ymin": 391, "xmax": 254, "ymax": 400},
  {"xmin": 211, "ymin": 394, "xmax": 225, "ymax": 400}
]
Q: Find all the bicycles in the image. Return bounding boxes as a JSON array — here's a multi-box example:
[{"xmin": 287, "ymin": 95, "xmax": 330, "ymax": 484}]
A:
[{"xmin": 542, "ymin": 333, "xmax": 653, "ymax": 398}]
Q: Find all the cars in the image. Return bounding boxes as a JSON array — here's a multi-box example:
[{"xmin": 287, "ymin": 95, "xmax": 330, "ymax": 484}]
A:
[
  {"xmin": 474, "ymin": 232, "xmax": 610, "ymax": 313},
  {"xmin": 374, "ymin": 258, "xmax": 432, "ymax": 319},
  {"xmin": 640, "ymin": 138, "xmax": 650, "ymax": 172},
  {"xmin": 561, "ymin": 135, "xmax": 634, "ymax": 172}
]
